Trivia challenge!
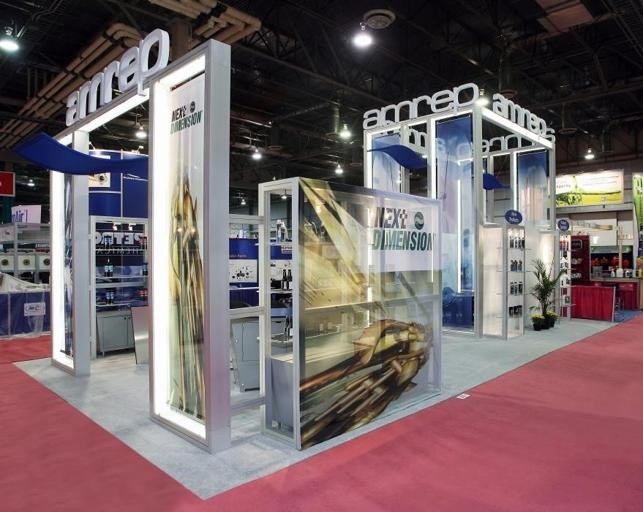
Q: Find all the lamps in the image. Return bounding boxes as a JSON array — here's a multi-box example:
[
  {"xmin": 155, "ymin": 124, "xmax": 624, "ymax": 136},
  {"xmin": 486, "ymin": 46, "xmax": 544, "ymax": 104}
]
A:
[
  {"xmin": 337, "ymin": 116, "xmax": 353, "ymax": 140},
  {"xmin": 585, "ymin": 145, "xmax": 595, "ymax": 161},
  {"xmin": 0, "ymin": 19, "xmax": 22, "ymax": 54},
  {"xmin": 135, "ymin": 121, "xmax": 147, "ymax": 140},
  {"xmin": 334, "ymin": 160, "xmax": 345, "ymax": 176},
  {"xmin": 252, "ymin": 144, "xmax": 262, "ymax": 160},
  {"xmin": 352, "ymin": 20, "xmax": 374, "ymax": 51}
]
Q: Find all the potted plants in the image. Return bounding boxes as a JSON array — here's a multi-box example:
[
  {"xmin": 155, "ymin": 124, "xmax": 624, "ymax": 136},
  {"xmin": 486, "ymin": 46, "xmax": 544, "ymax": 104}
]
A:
[{"xmin": 527, "ymin": 258, "xmax": 568, "ymax": 329}]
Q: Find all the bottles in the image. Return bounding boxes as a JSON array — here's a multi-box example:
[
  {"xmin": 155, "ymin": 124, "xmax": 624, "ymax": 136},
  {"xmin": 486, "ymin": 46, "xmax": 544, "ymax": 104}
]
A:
[
  {"xmin": 509, "ymin": 236, "xmax": 525, "ymax": 318},
  {"xmin": 277, "ymin": 220, "xmax": 293, "ymax": 339},
  {"xmin": 104, "ymin": 235, "xmax": 115, "ymax": 304}
]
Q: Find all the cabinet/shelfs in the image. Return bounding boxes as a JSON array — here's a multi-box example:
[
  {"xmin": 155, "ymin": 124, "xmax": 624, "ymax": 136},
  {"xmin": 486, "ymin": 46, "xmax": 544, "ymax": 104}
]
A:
[
  {"xmin": 256, "ymin": 176, "xmax": 444, "ymax": 452},
  {"xmin": 89, "ymin": 215, "xmax": 149, "ymax": 357},
  {"xmin": 572, "ymin": 278, "xmax": 642, "ymax": 322}
]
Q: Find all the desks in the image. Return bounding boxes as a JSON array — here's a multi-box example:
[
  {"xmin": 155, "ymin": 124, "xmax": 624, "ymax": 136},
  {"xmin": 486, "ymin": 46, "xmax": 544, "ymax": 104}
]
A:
[{"xmin": 230, "ymin": 302, "xmax": 287, "ymax": 393}]
